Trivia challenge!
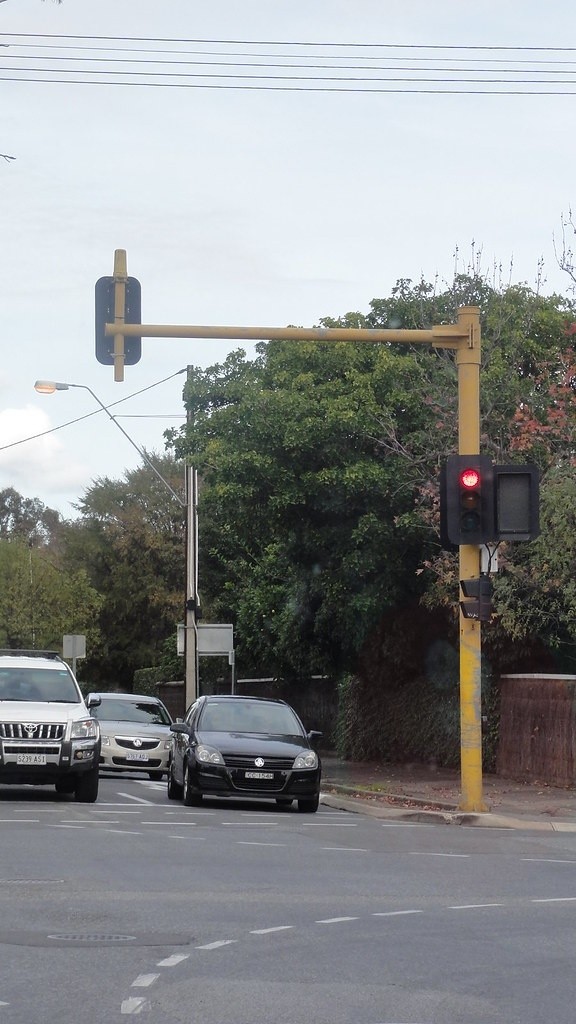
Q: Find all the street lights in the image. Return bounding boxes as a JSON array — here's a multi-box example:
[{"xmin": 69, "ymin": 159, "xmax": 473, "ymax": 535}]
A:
[{"xmin": 36, "ymin": 378, "xmax": 200, "ymax": 713}]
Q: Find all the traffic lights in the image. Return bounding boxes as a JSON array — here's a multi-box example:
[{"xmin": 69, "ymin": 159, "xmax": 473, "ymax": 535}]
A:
[
  {"xmin": 460, "ymin": 468, "xmax": 482, "ymax": 533},
  {"xmin": 460, "ymin": 577, "xmax": 479, "ymax": 618}
]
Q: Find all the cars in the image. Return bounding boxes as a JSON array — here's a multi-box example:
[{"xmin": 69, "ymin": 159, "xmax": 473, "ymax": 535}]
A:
[
  {"xmin": 165, "ymin": 695, "xmax": 323, "ymax": 813},
  {"xmin": 83, "ymin": 691, "xmax": 176, "ymax": 779}
]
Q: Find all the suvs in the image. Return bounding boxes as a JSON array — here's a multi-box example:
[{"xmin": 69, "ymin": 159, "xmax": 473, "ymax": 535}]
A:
[{"xmin": 0, "ymin": 649, "xmax": 101, "ymax": 803}]
